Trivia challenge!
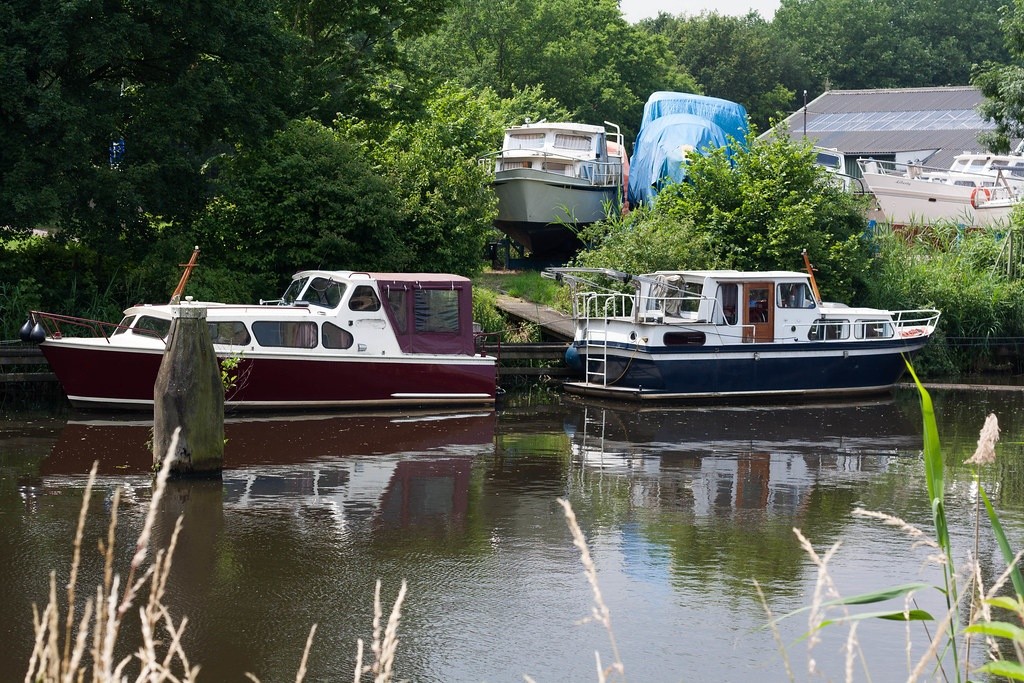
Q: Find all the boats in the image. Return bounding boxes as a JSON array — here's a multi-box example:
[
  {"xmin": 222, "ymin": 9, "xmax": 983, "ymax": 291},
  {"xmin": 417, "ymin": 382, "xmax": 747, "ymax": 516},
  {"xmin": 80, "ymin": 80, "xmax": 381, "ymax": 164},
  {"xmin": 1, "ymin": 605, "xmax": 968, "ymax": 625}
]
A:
[
  {"xmin": 554, "ymin": 246, "xmax": 941, "ymax": 399},
  {"xmin": 567, "ymin": 396, "xmax": 932, "ymax": 512},
  {"xmin": 18, "ymin": 245, "xmax": 507, "ymax": 417},
  {"xmin": 486, "ymin": 119, "xmax": 634, "ymax": 268},
  {"xmin": 37, "ymin": 408, "xmax": 495, "ymax": 518},
  {"xmin": 856, "ymin": 151, "xmax": 1024, "ymax": 253}
]
